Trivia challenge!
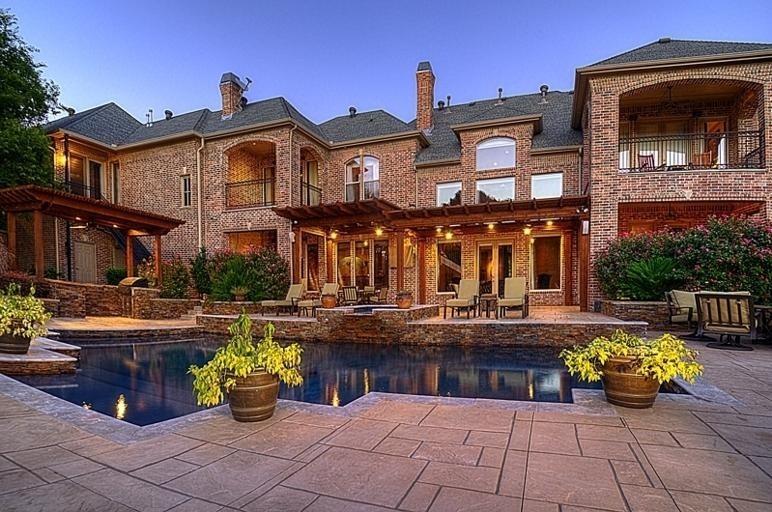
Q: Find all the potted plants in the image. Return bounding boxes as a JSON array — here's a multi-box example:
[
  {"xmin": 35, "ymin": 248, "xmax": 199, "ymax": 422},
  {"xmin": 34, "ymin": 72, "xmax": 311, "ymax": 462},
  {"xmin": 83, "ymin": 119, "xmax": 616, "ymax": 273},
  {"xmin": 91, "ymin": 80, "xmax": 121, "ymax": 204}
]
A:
[
  {"xmin": 557, "ymin": 327, "xmax": 705, "ymax": 409},
  {"xmin": 394, "ymin": 287, "xmax": 413, "ymax": 309},
  {"xmin": 0, "ymin": 280, "xmax": 53, "ymax": 355},
  {"xmin": 185, "ymin": 304, "xmax": 304, "ymax": 422}
]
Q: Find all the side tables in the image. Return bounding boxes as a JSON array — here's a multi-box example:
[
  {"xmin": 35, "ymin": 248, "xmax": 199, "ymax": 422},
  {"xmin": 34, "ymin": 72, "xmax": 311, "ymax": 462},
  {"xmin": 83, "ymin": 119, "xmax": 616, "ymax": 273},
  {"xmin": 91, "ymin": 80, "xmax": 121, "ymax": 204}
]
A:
[{"xmin": 480, "ymin": 298, "xmax": 497, "ymax": 320}]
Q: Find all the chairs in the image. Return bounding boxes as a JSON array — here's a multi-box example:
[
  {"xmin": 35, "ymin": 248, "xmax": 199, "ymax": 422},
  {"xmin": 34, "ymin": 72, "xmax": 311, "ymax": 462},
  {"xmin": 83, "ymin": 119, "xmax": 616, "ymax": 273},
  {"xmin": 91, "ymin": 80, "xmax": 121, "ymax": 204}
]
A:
[
  {"xmin": 664, "ymin": 290, "xmax": 717, "ymax": 342},
  {"xmin": 444, "ymin": 279, "xmax": 481, "ymax": 319},
  {"xmin": 495, "ymin": 276, "xmax": 529, "ymax": 319},
  {"xmin": 261, "ymin": 282, "xmax": 305, "ymax": 317},
  {"xmin": 695, "ymin": 292, "xmax": 761, "ymax": 351},
  {"xmin": 298, "ymin": 282, "xmax": 340, "ymax": 318},
  {"xmin": 638, "ymin": 154, "xmax": 666, "ymax": 172},
  {"xmin": 687, "ymin": 150, "xmax": 713, "ymax": 170},
  {"xmin": 319, "ymin": 284, "xmax": 388, "ymax": 306}
]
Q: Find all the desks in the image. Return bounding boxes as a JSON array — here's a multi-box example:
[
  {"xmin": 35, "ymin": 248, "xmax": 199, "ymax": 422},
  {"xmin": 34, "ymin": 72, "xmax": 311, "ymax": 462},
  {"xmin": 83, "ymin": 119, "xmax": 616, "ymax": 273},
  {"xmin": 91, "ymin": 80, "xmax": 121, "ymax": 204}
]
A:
[
  {"xmin": 754, "ymin": 305, "xmax": 772, "ymax": 344},
  {"xmin": 668, "ymin": 165, "xmax": 687, "ymax": 171}
]
